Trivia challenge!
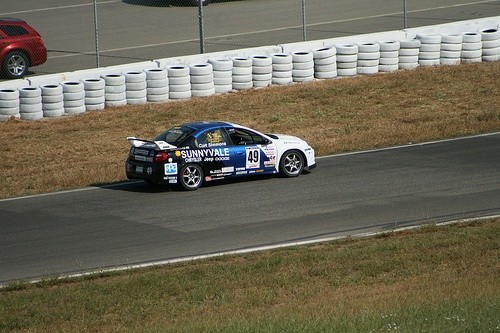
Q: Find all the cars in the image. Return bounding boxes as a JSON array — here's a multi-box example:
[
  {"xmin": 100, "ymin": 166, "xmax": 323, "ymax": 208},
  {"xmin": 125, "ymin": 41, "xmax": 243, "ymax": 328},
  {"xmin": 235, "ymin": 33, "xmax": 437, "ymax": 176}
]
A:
[{"xmin": 0, "ymin": 17, "xmax": 47, "ymax": 80}]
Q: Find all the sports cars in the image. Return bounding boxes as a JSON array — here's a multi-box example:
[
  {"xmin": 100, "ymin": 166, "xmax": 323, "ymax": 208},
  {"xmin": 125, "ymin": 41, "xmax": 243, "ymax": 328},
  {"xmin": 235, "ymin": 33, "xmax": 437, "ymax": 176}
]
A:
[{"xmin": 126, "ymin": 120, "xmax": 317, "ymax": 190}]
